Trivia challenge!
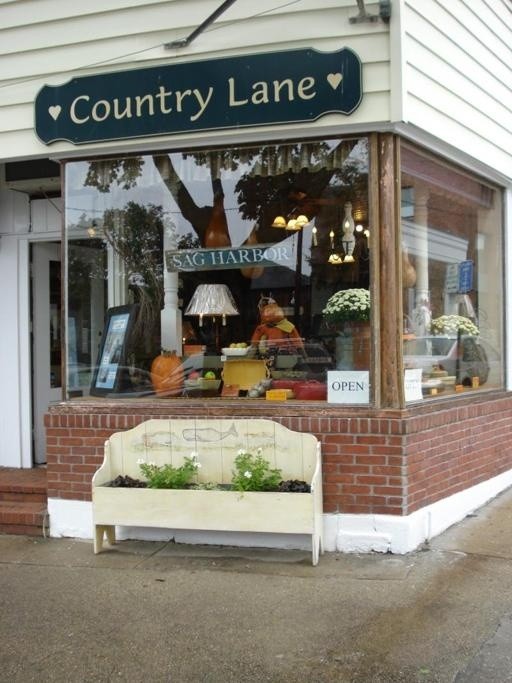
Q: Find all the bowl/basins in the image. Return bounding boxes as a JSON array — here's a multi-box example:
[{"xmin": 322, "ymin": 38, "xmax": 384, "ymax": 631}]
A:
[{"xmin": 183, "ymin": 379, "xmax": 222, "ymax": 397}]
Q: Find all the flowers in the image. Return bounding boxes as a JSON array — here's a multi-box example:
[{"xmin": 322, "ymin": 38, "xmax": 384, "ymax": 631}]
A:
[
  {"xmin": 430, "ymin": 314, "xmax": 480, "ymax": 336},
  {"xmin": 322, "ymin": 288, "xmax": 370, "ymax": 336}
]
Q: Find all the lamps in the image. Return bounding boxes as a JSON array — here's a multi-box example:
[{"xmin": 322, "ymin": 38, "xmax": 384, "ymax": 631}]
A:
[{"xmin": 184, "ymin": 284, "xmax": 240, "ymax": 354}]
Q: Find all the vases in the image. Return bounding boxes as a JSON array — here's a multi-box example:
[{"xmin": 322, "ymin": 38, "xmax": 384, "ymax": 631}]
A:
[{"xmin": 335, "ymin": 337, "xmax": 360, "ymax": 370}]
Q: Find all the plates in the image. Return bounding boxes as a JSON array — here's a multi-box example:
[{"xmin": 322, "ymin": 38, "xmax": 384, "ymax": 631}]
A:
[{"xmin": 221, "ymin": 347, "xmax": 250, "ymax": 357}]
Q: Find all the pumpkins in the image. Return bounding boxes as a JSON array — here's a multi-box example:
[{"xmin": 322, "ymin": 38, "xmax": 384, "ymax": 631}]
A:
[
  {"xmin": 151, "ymin": 350, "xmax": 183, "ymax": 398},
  {"xmin": 260, "ymin": 302, "xmax": 284, "ymax": 324}
]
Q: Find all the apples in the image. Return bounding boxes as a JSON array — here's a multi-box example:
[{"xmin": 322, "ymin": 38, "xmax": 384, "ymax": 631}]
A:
[{"xmin": 205, "ymin": 371, "xmax": 215, "ymax": 380}]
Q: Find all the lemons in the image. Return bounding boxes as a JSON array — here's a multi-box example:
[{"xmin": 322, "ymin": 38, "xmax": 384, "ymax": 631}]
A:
[
  {"xmin": 242, "ymin": 343, "xmax": 248, "ymax": 348},
  {"xmin": 236, "ymin": 343, "xmax": 242, "ymax": 348},
  {"xmin": 229, "ymin": 343, "xmax": 236, "ymax": 348}
]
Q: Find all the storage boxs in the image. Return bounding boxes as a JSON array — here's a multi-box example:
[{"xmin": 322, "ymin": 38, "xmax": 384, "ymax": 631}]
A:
[{"xmin": 184, "ymin": 345, "xmax": 210, "ymax": 356}]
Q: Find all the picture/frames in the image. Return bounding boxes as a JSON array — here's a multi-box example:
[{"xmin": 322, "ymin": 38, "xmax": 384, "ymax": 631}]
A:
[{"xmin": 89, "ymin": 304, "xmax": 140, "ymax": 397}]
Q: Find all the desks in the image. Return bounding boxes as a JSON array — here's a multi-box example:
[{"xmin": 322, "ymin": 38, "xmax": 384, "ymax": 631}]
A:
[{"xmin": 191, "ymin": 355, "xmax": 301, "ymax": 376}]
